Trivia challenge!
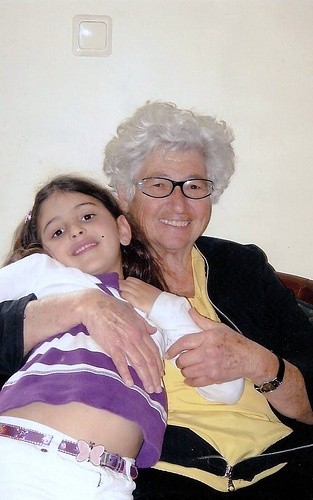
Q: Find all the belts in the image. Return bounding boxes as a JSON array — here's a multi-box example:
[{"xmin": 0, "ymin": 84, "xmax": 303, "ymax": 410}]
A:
[{"xmin": 1, "ymin": 420, "xmax": 140, "ymax": 479}]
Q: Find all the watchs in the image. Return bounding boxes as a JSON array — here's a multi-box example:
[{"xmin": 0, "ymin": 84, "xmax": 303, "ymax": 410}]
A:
[{"xmin": 254, "ymin": 352, "xmax": 285, "ymax": 394}]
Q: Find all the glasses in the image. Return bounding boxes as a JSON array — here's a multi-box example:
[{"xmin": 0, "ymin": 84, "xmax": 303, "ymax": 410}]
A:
[{"xmin": 136, "ymin": 176, "xmax": 214, "ymax": 200}]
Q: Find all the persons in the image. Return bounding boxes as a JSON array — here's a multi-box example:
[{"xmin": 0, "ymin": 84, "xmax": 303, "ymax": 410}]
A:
[
  {"xmin": 0, "ymin": 178, "xmax": 244, "ymax": 500},
  {"xmin": 0, "ymin": 100, "xmax": 313, "ymax": 500}
]
